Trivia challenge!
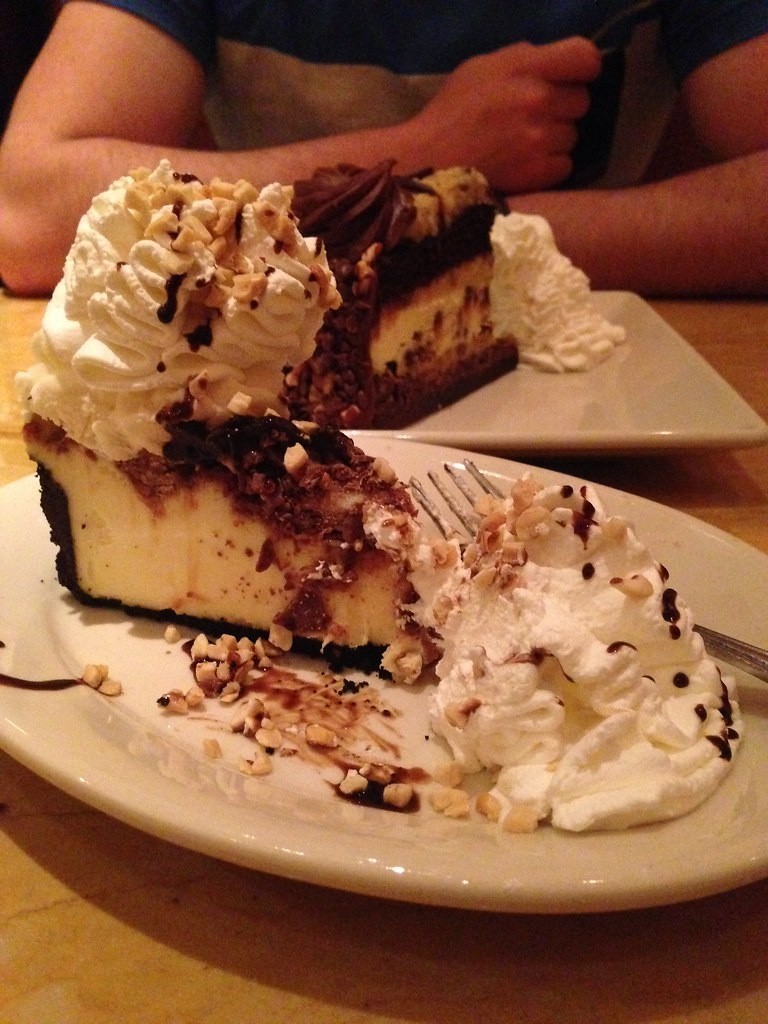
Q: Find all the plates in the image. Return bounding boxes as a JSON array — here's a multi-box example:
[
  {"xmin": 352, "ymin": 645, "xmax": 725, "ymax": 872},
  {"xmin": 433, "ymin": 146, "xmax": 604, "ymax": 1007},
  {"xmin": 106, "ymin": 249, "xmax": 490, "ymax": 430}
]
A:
[
  {"xmin": 340, "ymin": 291, "xmax": 768, "ymax": 453},
  {"xmin": 0, "ymin": 435, "xmax": 768, "ymax": 914}
]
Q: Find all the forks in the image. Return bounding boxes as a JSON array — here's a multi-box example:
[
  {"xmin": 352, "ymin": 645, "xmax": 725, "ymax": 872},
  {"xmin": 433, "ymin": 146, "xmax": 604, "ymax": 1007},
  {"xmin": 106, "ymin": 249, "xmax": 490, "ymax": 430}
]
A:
[{"xmin": 406, "ymin": 457, "xmax": 768, "ymax": 688}]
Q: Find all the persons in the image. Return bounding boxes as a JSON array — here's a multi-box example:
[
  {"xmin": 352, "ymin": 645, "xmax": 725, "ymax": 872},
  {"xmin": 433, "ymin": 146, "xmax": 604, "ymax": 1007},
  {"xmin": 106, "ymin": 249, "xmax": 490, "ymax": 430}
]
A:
[{"xmin": 0, "ymin": 0, "xmax": 768, "ymax": 302}]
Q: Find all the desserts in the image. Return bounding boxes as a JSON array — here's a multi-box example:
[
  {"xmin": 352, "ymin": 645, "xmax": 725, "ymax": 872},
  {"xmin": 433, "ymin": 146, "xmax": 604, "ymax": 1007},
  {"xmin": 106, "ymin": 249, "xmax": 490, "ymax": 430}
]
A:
[{"xmin": 18, "ymin": 157, "xmax": 743, "ymax": 830}]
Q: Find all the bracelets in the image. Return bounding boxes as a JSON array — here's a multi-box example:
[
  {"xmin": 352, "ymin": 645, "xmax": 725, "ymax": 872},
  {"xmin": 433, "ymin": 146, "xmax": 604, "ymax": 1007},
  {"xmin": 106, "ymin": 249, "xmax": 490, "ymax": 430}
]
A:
[{"xmin": 498, "ymin": 196, "xmax": 509, "ymax": 215}]
